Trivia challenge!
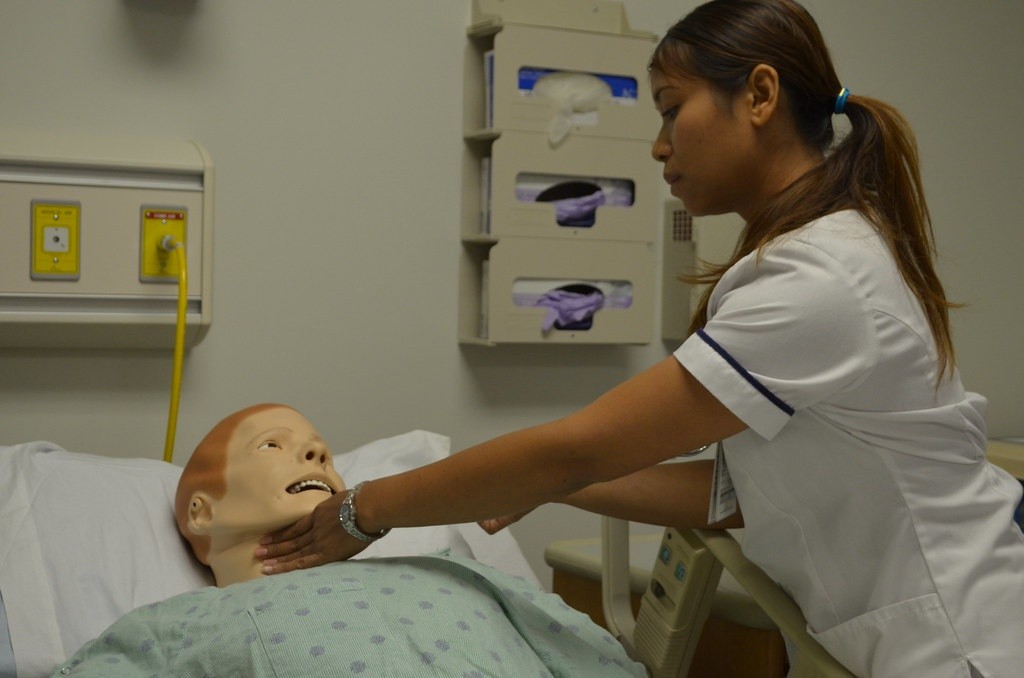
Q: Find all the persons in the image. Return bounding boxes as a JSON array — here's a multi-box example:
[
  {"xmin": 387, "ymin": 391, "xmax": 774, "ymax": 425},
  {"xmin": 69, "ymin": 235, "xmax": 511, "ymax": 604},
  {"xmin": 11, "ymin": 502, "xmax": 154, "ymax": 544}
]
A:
[
  {"xmin": 49, "ymin": 404, "xmax": 649, "ymax": 678},
  {"xmin": 253, "ymin": 0, "xmax": 1024, "ymax": 678}
]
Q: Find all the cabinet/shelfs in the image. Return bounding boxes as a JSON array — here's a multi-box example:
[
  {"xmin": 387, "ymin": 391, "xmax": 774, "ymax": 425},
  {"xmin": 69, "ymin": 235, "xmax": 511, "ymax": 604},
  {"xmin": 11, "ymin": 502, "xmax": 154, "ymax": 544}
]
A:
[{"xmin": 455, "ymin": 0, "xmax": 665, "ymax": 348}]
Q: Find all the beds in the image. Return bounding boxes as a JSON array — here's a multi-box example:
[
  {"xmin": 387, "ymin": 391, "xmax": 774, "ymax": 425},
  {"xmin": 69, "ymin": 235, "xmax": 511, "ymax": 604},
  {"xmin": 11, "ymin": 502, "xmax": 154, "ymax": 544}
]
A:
[{"xmin": 451, "ymin": 513, "xmax": 855, "ymax": 678}]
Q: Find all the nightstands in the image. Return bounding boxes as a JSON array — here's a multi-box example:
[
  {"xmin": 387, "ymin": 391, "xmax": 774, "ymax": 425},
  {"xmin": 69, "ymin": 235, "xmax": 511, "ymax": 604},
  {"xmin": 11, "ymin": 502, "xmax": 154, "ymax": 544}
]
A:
[{"xmin": 544, "ymin": 527, "xmax": 791, "ymax": 678}]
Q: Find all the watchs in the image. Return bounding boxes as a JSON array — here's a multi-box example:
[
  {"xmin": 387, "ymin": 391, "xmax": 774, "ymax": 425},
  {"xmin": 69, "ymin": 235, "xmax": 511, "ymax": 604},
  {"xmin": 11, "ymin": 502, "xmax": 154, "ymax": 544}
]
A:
[{"xmin": 340, "ymin": 481, "xmax": 392, "ymax": 543}]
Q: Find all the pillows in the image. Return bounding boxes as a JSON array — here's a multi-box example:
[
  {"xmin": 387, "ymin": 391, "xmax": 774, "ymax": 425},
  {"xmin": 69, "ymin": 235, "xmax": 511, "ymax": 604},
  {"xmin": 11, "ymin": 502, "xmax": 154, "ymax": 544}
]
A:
[{"xmin": 0, "ymin": 429, "xmax": 453, "ymax": 678}]
